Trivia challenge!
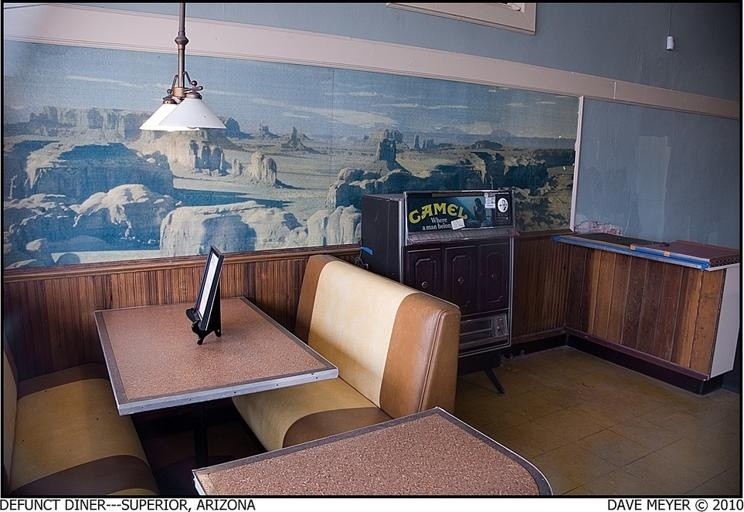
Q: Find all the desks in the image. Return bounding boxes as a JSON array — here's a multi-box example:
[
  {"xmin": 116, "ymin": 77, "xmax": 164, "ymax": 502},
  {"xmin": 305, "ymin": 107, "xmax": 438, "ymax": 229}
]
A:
[{"xmin": 92, "ymin": 295, "xmax": 338, "ymax": 500}]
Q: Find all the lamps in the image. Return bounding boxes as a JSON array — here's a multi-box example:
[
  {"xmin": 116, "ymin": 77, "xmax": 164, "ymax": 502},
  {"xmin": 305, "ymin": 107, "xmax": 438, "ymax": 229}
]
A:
[{"xmin": 139, "ymin": 2, "xmax": 229, "ymax": 134}]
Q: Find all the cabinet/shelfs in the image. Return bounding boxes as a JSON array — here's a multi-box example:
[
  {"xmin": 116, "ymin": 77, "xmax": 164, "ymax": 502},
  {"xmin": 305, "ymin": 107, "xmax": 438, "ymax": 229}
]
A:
[{"xmin": 359, "ymin": 186, "xmax": 520, "ymax": 393}]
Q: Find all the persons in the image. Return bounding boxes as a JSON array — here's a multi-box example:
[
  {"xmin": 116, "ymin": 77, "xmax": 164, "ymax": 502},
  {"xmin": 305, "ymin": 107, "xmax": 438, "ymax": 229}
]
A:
[{"xmin": 473, "ymin": 198, "xmax": 486, "ymax": 223}]
